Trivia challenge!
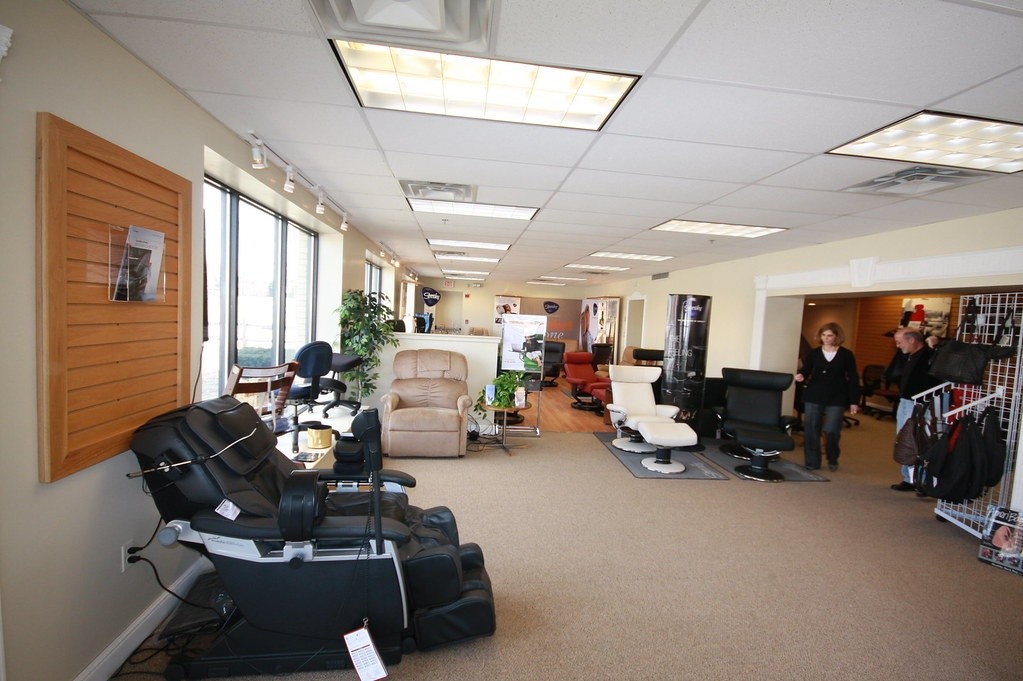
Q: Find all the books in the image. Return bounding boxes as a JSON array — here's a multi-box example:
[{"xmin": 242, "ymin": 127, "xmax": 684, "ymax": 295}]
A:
[{"xmin": 292, "ymin": 452, "xmax": 324, "ymax": 462}]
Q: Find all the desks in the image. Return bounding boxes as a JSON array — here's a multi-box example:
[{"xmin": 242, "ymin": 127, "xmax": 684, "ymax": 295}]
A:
[{"xmin": 475, "ymin": 400, "xmax": 529, "ymax": 457}]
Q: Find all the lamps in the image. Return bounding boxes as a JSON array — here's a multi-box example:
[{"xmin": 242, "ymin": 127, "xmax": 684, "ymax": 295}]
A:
[
  {"xmin": 250, "ymin": 138, "xmax": 270, "ymax": 173},
  {"xmin": 283, "ymin": 164, "xmax": 298, "ymax": 192},
  {"xmin": 340, "ymin": 211, "xmax": 350, "ymax": 230},
  {"xmin": 380, "ymin": 249, "xmax": 419, "ymax": 280},
  {"xmin": 309, "ymin": 184, "xmax": 327, "ymax": 215}
]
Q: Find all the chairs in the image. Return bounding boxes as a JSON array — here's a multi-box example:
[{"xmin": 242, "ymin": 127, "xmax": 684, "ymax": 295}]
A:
[
  {"xmin": 269, "ymin": 340, "xmax": 340, "ymax": 452},
  {"xmin": 524, "ymin": 341, "xmax": 799, "ymax": 482},
  {"xmin": 384, "ymin": 318, "xmax": 405, "ymax": 333},
  {"xmin": 854, "ymin": 363, "xmax": 884, "ymax": 412},
  {"xmin": 305, "ymin": 352, "xmax": 364, "ymax": 419},
  {"xmin": 468, "ymin": 327, "xmax": 490, "ymax": 336}
]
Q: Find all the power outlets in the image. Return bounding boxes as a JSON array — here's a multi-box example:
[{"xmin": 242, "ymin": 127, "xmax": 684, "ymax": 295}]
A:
[{"xmin": 120, "ymin": 537, "xmax": 136, "ymax": 574}]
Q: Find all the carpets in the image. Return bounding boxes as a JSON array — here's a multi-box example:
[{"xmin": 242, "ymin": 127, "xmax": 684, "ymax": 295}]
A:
[
  {"xmin": 694, "ymin": 437, "xmax": 832, "ymax": 484},
  {"xmin": 595, "ymin": 431, "xmax": 729, "ymax": 480}
]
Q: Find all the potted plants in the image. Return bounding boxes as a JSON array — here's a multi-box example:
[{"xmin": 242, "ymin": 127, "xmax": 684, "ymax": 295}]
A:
[{"xmin": 474, "ymin": 373, "xmax": 531, "ymax": 419}]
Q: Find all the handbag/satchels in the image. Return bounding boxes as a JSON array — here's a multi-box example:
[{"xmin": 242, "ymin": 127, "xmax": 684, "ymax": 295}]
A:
[
  {"xmin": 913, "ymin": 404, "xmax": 1006, "ymax": 504},
  {"xmin": 929, "ymin": 299, "xmax": 1020, "ymax": 385}
]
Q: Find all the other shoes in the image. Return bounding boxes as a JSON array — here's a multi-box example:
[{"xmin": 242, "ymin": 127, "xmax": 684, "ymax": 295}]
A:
[
  {"xmin": 828, "ymin": 462, "xmax": 838, "ymax": 471},
  {"xmin": 806, "ymin": 466, "xmax": 819, "ymax": 469},
  {"xmin": 891, "ymin": 482, "xmax": 918, "ymax": 490}
]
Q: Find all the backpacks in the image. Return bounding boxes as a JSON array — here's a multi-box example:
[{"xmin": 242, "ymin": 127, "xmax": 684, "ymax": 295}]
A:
[{"xmin": 893, "ymin": 404, "xmax": 936, "ymax": 466}]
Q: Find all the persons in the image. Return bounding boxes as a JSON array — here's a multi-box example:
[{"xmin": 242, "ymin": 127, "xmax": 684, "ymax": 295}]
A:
[
  {"xmin": 522, "ymin": 336, "xmax": 542, "ymax": 366},
  {"xmin": 889, "ymin": 327, "xmax": 943, "ymax": 498},
  {"xmin": 793, "ymin": 322, "xmax": 861, "ymax": 472},
  {"xmin": 503, "ymin": 304, "xmax": 516, "ymax": 314}
]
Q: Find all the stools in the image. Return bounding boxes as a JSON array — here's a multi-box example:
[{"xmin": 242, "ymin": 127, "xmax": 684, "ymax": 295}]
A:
[
  {"xmin": 585, "ymin": 383, "xmax": 608, "ymax": 418},
  {"xmin": 638, "ymin": 424, "xmax": 697, "ymax": 473}
]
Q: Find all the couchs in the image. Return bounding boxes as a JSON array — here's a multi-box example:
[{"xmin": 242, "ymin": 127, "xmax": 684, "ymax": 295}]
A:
[
  {"xmin": 126, "ymin": 392, "xmax": 497, "ymax": 669},
  {"xmin": 380, "ymin": 350, "xmax": 471, "ymax": 459}
]
